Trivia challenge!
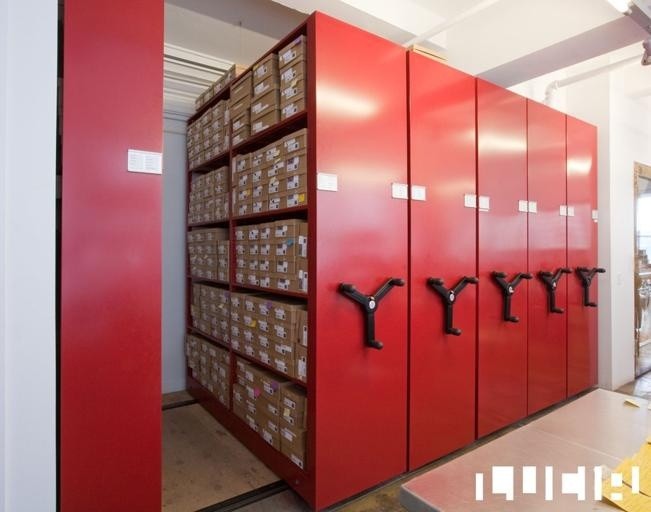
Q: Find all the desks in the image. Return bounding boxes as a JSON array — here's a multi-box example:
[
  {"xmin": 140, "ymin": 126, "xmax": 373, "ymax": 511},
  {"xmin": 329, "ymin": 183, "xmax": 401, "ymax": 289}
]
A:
[
  {"xmin": 400, "ymin": 425, "xmax": 651, "ymax": 512},
  {"xmin": 526, "ymin": 389, "xmax": 651, "ymax": 461}
]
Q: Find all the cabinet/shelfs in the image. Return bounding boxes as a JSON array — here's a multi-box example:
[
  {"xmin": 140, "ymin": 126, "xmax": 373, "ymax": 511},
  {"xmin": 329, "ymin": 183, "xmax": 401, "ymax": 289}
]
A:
[{"xmin": 59, "ymin": 0, "xmax": 165, "ymax": 512}]
